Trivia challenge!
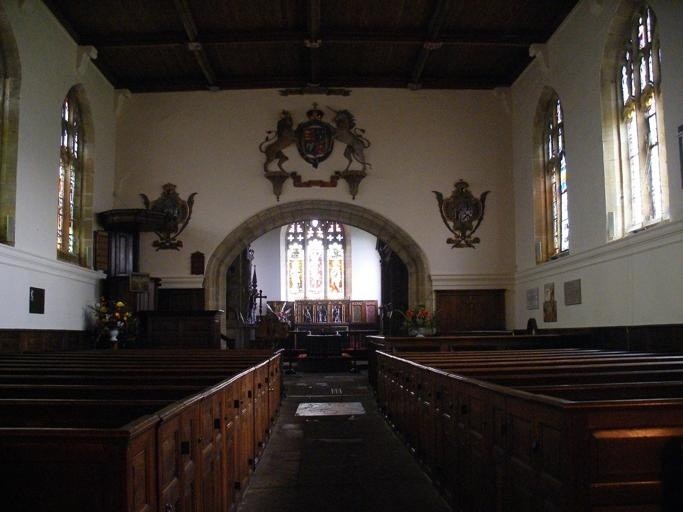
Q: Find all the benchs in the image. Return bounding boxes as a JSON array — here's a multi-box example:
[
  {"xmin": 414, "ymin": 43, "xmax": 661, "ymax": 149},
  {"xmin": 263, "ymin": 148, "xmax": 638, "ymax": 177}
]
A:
[
  {"xmin": 375, "ymin": 347, "xmax": 683, "ymax": 511},
  {"xmin": 0, "ymin": 348, "xmax": 287, "ymax": 511}
]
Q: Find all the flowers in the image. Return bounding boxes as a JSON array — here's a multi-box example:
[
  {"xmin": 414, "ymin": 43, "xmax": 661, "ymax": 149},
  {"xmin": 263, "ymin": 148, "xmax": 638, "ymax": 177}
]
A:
[
  {"xmin": 86, "ymin": 294, "xmax": 139, "ymax": 343},
  {"xmin": 394, "ymin": 304, "xmax": 443, "ymax": 334}
]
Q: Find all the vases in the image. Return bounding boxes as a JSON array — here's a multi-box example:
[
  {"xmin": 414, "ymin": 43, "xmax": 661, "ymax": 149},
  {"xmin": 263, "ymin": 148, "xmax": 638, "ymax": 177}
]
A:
[
  {"xmin": 110, "ymin": 328, "xmax": 119, "ymax": 341},
  {"xmin": 415, "ymin": 328, "xmax": 425, "ymax": 337}
]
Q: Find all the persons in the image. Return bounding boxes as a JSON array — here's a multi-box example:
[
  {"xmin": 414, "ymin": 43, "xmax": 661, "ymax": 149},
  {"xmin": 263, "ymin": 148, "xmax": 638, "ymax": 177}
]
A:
[
  {"xmin": 332, "ymin": 308, "xmax": 339, "ymax": 322},
  {"xmin": 304, "ymin": 308, "xmax": 311, "ymax": 321},
  {"xmin": 317, "ymin": 310, "xmax": 322, "ymax": 322}
]
